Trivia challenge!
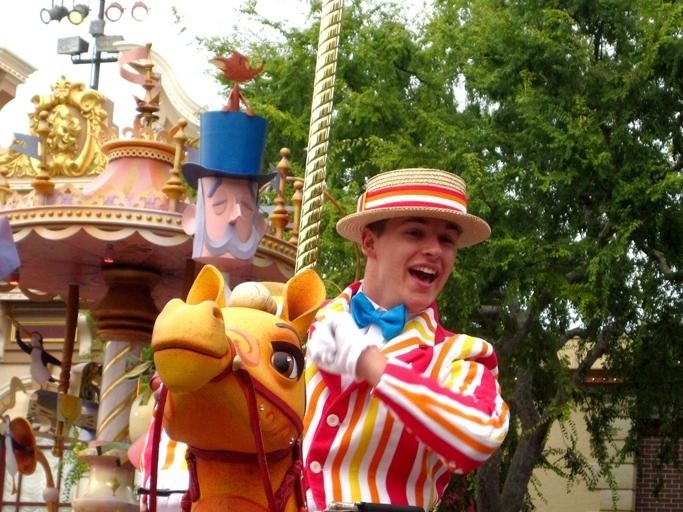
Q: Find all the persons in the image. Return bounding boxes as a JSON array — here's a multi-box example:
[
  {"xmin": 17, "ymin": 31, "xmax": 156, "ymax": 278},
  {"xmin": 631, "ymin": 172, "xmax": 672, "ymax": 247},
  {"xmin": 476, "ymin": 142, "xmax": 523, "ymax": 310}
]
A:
[{"xmin": 290, "ymin": 165, "xmax": 510, "ymax": 512}]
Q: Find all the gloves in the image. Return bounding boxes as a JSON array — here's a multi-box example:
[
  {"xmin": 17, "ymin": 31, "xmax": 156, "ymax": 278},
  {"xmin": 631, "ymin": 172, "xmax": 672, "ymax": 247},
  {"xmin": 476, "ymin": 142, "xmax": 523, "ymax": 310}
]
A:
[{"xmin": 304, "ymin": 308, "xmax": 377, "ymax": 385}]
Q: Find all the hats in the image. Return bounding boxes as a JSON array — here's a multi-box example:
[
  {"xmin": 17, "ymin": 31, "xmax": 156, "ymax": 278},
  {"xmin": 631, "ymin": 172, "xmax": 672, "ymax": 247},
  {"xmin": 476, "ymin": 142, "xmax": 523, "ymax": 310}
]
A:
[{"xmin": 334, "ymin": 167, "xmax": 494, "ymax": 250}]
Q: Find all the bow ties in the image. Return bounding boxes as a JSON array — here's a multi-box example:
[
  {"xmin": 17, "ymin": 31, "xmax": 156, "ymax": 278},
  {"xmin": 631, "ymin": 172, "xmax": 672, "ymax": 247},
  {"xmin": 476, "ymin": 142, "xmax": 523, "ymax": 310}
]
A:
[{"xmin": 348, "ymin": 291, "xmax": 408, "ymax": 342}]
